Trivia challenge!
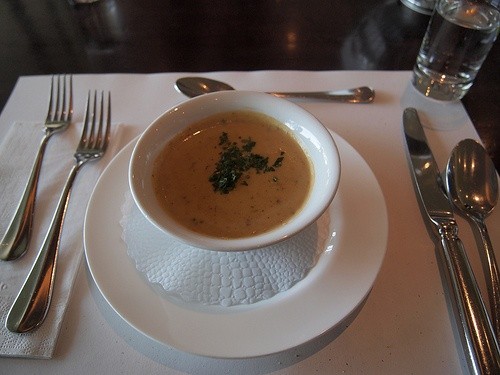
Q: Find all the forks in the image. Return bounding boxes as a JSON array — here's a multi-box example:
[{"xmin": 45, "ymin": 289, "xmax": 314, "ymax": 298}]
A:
[
  {"xmin": 4, "ymin": 87, "xmax": 111, "ymax": 333},
  {"xmin": 0, "ymin": 72, "xmax": 73, "ymax": 260}
]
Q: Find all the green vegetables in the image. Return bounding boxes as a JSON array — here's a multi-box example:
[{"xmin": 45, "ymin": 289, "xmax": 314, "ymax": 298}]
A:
[{"xmin": 208, "ymin": 132, "xmax": 286, "ymax": 196}]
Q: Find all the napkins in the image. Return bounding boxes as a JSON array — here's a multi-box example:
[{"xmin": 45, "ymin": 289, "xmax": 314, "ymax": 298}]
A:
[{"xmin": 0, "ymin": 120, "xmax": 122, "ymax": 358}]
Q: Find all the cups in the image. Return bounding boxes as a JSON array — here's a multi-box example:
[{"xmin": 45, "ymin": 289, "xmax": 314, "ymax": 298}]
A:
[{"xmin": 410, "ymin": 0, "xmax": 500, "ymax": 105}]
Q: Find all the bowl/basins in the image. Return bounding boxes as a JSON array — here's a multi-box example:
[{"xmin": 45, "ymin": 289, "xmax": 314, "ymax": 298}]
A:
[{"xmin": 127, "ymin": 88, "xmax": 341, "ymax": 249}]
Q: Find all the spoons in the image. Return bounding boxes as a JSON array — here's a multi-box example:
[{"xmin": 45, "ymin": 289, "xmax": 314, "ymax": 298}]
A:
[
  {"xmin": 173, "ymin": 76, "xmax": 375, "ymax": 104},
  {"xmin": 444, "ymin": 138, "xmax": 500, "ymax": 340}
]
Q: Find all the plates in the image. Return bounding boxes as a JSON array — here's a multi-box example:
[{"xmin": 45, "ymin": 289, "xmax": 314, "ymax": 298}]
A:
[{"xmin": 83, "ymin": 127, "xmax": 389, "ymax": 359}]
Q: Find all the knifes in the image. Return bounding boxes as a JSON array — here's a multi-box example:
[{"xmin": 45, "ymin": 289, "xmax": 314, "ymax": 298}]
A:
[{"xmin": 401, "ymin": 106, "xmax": 500, "ymax": 375}]
